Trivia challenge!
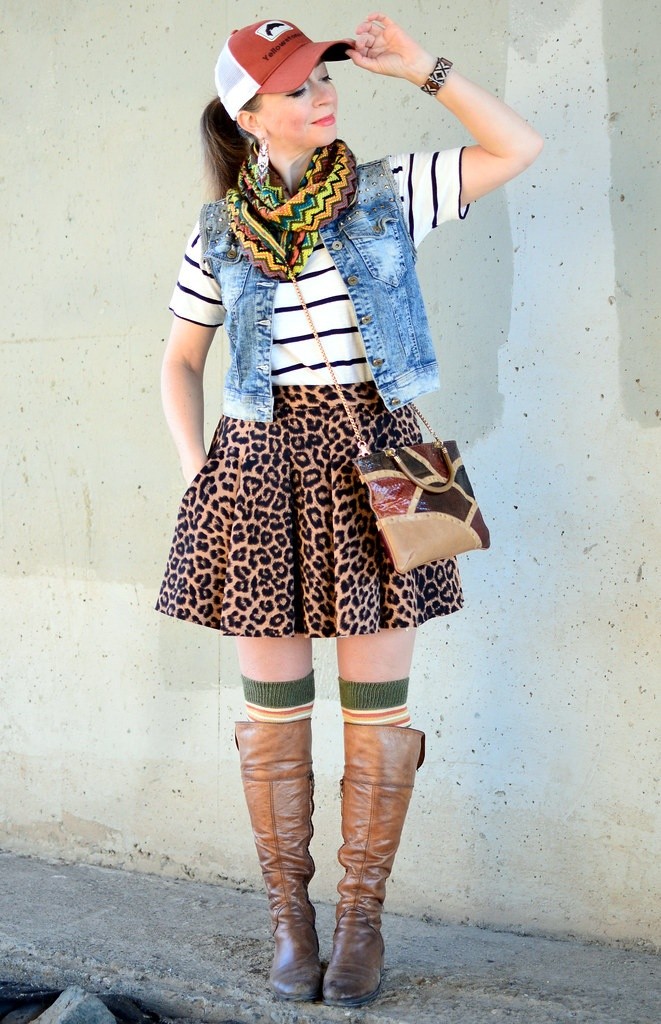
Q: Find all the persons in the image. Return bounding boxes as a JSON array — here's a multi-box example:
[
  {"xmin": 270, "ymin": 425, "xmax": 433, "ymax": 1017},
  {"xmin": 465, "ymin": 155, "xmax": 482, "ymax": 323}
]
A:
[{"xmin": 152, "ymin": 12, "xmax": 545, "ymax": 1007}]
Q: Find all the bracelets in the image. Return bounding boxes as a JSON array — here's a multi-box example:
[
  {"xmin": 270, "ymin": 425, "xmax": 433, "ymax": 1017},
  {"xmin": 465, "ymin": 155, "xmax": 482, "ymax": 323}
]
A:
[{"xmin": 419, "ymin": 56, "xmax": 453, "ymax": 95}]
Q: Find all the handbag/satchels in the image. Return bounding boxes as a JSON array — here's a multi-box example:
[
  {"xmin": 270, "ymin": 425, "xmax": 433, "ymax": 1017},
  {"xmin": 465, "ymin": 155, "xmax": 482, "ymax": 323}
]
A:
[{"xmin": 351, "ymin": 440, "xmax": 492, "ymax": 575}]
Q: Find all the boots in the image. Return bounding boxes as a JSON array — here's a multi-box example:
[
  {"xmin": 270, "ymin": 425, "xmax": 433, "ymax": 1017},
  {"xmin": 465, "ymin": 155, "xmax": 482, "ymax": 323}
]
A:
[
  {"xmin": 234, "ymin": 717, "xmax": 321, "ymax": 1002},
  {"xmin": 323, "ymin": 722, "xmax": 426, "ymax": 1007}
]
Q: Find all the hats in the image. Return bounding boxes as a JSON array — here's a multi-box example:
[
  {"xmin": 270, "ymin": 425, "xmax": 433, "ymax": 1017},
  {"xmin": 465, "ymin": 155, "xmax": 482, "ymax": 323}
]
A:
[{"xmin": 214, "ymin": 20, "xmax": 354, "ymax": 121}]
